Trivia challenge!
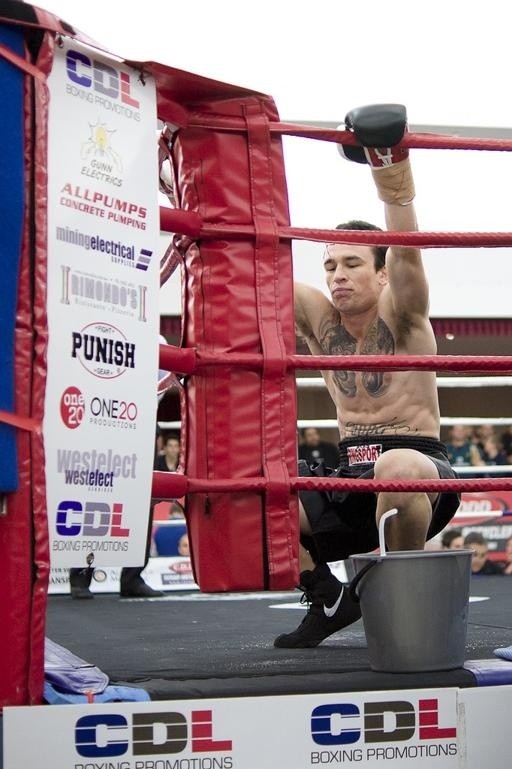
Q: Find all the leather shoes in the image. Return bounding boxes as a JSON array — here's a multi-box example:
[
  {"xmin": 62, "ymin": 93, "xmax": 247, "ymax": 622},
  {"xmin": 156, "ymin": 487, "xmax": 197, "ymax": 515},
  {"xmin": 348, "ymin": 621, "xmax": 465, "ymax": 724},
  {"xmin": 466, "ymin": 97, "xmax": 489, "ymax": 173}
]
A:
[
  {"xmin": 70, "ymin": 586, "xmax": 94, "ymax": 600},
  {"xmin": 121, "ymin": 584, "xmax": 163, "ymax": 598}
]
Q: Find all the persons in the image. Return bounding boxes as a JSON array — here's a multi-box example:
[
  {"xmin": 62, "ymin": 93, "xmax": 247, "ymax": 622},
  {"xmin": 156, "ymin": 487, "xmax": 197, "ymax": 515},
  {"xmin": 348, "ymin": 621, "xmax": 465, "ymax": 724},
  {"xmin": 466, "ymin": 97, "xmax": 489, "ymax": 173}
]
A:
[
  {"xmin": 70, "ymin": 333, "xmax": 170, "ymax": 601},
  {"xmin": 147, "ymin": 424, "xmax": 511, "ymax": 580},
  {"xmin": 157, "ymin": 100, "xmax": 464, "ymax": 653}
]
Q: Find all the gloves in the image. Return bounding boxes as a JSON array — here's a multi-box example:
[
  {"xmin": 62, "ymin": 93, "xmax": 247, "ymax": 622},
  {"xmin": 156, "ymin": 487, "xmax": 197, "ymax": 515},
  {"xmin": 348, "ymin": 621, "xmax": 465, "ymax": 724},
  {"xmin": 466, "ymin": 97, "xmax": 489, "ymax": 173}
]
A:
[
  {"xmin": 337, "ymin": 105, "xmax": 416, "ymax": 208},
  {"xmin": 156, "ymin": 131, "xmax": 176, "ymax": 204}
]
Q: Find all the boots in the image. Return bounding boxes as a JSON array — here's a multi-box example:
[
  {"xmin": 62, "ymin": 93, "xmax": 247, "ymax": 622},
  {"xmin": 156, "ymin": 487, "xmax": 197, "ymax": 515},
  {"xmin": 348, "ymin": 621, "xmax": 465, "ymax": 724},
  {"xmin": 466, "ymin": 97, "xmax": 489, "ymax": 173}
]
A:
[{"xmin": 274, "ymin": 564, "xmax": 360, "ymax": 649}]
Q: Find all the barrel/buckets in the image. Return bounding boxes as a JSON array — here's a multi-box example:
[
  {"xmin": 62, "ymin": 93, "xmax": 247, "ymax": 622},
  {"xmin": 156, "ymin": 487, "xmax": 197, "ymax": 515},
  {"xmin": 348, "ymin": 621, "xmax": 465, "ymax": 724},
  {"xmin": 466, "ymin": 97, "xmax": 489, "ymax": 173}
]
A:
[{"xmin": 349, "ymin": 549, "xmax": 475, "ymax": 674}]
